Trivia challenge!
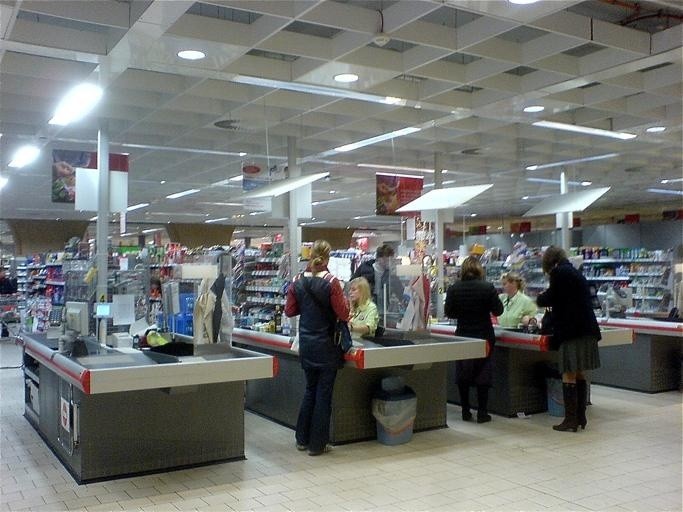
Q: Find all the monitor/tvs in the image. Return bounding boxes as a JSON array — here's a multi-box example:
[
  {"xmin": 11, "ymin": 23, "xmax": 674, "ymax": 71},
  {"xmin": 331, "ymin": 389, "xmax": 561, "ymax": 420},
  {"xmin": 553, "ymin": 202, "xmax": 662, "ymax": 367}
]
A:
[
  {"xmin": 610, "ymin": 286, "xmax": 633, "ymax": 308},
  {"xmin": 64, "ymin": 301, "xmax": 89, "ymax": 336}
]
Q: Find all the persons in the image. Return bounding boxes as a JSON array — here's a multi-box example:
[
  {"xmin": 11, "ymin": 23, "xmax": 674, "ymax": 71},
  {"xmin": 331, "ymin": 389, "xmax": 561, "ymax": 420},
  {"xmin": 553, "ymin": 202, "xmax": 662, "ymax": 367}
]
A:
[
  {"xmin": 346, "ymin": 275, "xmax": 380, "ymax": 339},
  {"xmin": 52, "ymin": 149, "xmax": 91, "ymax": 177},
  {"xmin": 536, "ymin": 244, "xmax": 603, "ymax": 432},
  {"xmin": 498, "ymin": 271, "xmax": 537, "ymax": 330},
  {"xmin": 0, "ymin": 266, "xmax": 12, "ymax": 337},
  {"xmin": 284, "ymin": 239, "xmax": 350, "ymax": 457},
  {"xmin": 444, "ymin": 255, "xmax": 504, "ymax": 423},
  {"xmin": 376, "ymin": 175, "xmax": 401, "ymax": 195},
  {"xmin": 351, "ymin": 245, "xmax": 404, "ymax": 326}
]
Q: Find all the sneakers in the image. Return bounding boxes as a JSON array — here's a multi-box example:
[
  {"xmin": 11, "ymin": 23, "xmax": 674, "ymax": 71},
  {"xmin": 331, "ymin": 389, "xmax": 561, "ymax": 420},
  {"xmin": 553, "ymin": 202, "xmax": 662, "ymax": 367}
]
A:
[
  {"xmin": 296, "ymin": 443, "xmax": 307, "ymax": 451},
  {"xmin": 307, "ymin": 444, "xmax": 333, "ymax": 456}
]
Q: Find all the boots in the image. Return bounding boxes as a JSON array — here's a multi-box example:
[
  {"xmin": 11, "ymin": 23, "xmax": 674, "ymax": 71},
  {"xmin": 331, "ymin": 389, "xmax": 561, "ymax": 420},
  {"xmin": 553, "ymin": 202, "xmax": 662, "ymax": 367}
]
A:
[
  {"xmin": 477, "ymin": 385, "xmax": 491, "ymax": 423},
  {"xmin": 577, "ymin": 380, "xmax": 586, "ymax": 429},
  {"xmin": 553, "ymin": 382, "xmax": 577, "ymax": 432},
  {"xmin": 462, "ymin": 386, "xmax": 473, "ymax": 421}
]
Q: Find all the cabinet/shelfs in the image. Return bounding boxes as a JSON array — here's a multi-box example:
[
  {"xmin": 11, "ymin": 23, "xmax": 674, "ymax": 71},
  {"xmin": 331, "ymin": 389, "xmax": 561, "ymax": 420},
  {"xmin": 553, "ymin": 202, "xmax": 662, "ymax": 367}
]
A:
[
  {"xmin": 431, "ymin": 324, "xmax": 636, "ymax": 417},
  {"xmin": 220, "ymin": 327, "xmax": 490, "ymax": 447},
  {"xmin": 19, "ymin": 331, "xmax": 280, "ymax": 486},
  {"xmin": 592, "ymin": 317, "xmax": 683, "ymax": 393}
]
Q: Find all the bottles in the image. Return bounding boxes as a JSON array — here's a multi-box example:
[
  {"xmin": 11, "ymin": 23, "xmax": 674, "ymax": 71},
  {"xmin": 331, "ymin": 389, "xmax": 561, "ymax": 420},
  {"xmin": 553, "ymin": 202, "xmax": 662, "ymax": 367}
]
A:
[{"xmin": 247, "ymin": 305, "xmax": 290, "ymax": 336}]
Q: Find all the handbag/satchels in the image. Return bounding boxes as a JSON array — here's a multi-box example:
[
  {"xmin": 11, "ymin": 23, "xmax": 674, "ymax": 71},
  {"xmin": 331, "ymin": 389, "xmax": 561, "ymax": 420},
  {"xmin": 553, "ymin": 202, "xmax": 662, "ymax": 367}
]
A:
[
  {"xmin": 330, "ymin": 320, "xmax": 352, "ymax": 353},
  {"xmin": 542, "ymin": 310, "xmax": 554, "ymax": 334}
]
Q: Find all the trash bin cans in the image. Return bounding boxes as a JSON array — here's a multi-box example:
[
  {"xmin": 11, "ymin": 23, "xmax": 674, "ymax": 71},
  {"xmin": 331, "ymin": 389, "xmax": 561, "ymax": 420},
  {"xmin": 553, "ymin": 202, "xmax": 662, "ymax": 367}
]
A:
[
  {"xmin": 372, "ymin": 385, "xmax": 418, "ymax": 445},
  {"xmin": 546, "ymin": 378, "xmax": 569, "ymax": 417}
]
